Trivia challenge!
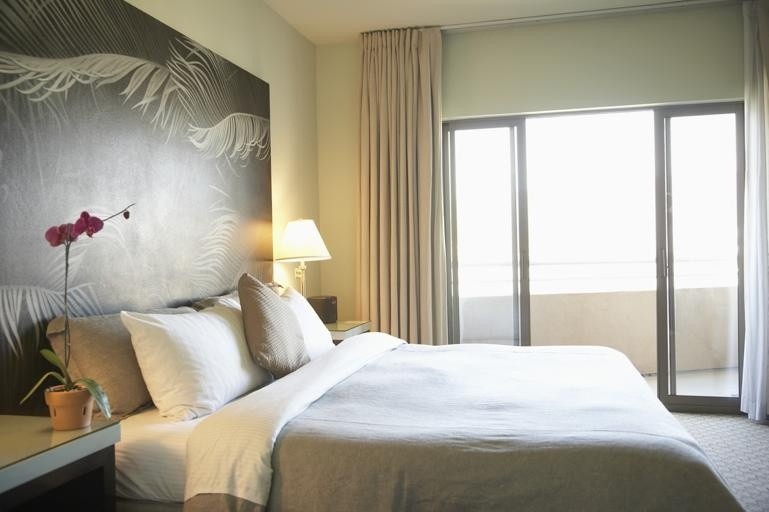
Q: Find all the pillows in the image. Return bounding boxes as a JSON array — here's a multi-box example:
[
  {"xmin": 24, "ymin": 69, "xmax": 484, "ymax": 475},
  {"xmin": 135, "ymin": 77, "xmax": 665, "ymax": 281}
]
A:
[
  {"xmin": 281, "ymin": 286, "xmax": 333, "ymax": 361},
  {"xmin": 47, "ymin": 305, "xmax": 199, "ymax": 422},
  {"xmin": 193, "ymin": 282, "xmax": 278, "ymax": 312},
  {"xmin": 238, "ymin": 271, "xmax": 310, "ymax": 376},
  {"xmin": 120, "ymin": 296, "xmax": 275, "ymax": 420}
]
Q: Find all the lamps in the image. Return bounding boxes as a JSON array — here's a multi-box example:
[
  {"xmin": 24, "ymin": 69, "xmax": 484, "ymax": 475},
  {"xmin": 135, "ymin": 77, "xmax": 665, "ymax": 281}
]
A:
[{"xmin": 273, "ymin": 216, "xmax": 329, "ymax": 299}]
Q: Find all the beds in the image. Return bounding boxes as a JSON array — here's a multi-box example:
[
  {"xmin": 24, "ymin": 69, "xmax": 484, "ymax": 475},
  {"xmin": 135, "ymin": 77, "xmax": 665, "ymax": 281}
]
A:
[{"xmin": 115, "ymin": 333, "xmax": 713, "ymax": 512}]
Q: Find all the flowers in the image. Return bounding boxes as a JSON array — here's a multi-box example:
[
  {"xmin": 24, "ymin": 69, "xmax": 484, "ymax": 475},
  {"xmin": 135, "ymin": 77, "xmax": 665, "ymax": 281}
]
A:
[{"xmin": 44, "ymin": 199, "xmax": 135, "ymax": 386}]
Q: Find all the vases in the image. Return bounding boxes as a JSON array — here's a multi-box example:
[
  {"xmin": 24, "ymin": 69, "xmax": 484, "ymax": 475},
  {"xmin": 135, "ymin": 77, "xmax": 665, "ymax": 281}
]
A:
[{"xmin": 43, "ymin": 386, "xmax": 96, "ymax": 430}]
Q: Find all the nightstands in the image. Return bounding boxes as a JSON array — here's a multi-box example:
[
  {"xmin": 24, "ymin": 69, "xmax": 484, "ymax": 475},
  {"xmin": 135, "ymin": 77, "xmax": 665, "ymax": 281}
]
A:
[
  {"xmin": 325, "ymin": 320, "xmax": 370, "ymax": 344},
  {"xmin": 0, "ymin": 412, "xmax": 120, "ymax": 512}
]
